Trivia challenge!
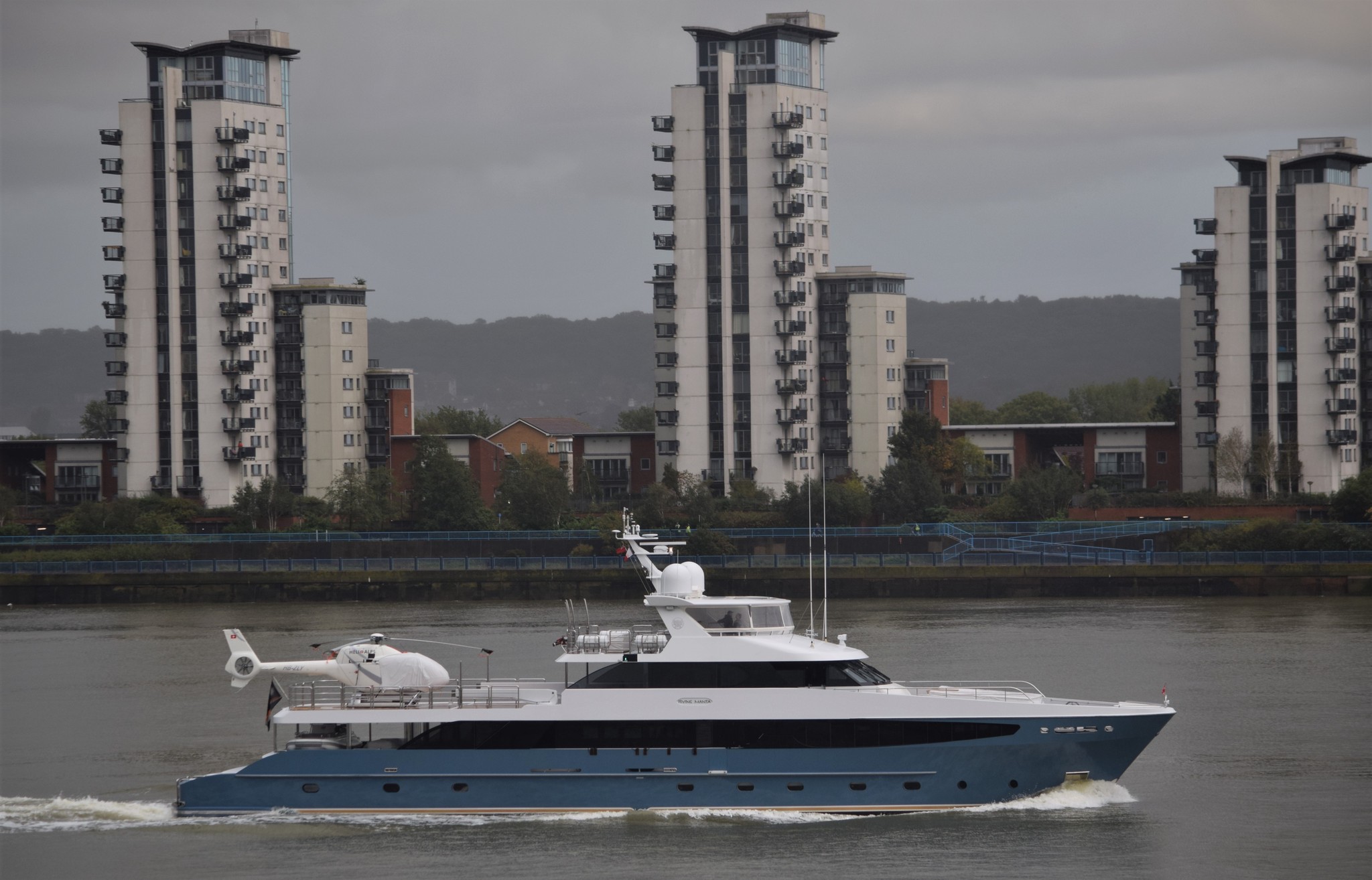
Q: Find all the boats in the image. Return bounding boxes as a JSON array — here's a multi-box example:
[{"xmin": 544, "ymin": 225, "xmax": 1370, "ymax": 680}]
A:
[
  {"xmin": 171, "ymin": 507, "xmax": 1179, "ymax": 824},
  {"xmin": 285, "ymin": 724, "xmax": 365, "ymax": 750}
]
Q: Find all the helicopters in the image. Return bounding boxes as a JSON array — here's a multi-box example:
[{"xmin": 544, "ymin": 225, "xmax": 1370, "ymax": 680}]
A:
[{"xmin": 221, "ymin": 627, "xmax": 494, "ymax": 708}]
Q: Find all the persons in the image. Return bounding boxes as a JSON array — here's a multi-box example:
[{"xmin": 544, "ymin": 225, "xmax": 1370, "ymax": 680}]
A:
[
  {"xmin": 716, "ymin": 611, "xmax": 735, "ymax": 636},
  {"xmin": 685, "ymin": 523, "xmax": 691, "ymax": 536},
  {"xmin": 734, "ymin": 613, "xmax": 743, "ymax": 636},
  {"xmin": 911, "ymin": 521, "xmax": 921, "ymax": 535},
  {"xmin": 814, "ymin": 523, "xmax": 822, "ymax": 536},
  {"xmin": 674, "ymin": 522, "xmax": 681, "ymax": 536}
]
